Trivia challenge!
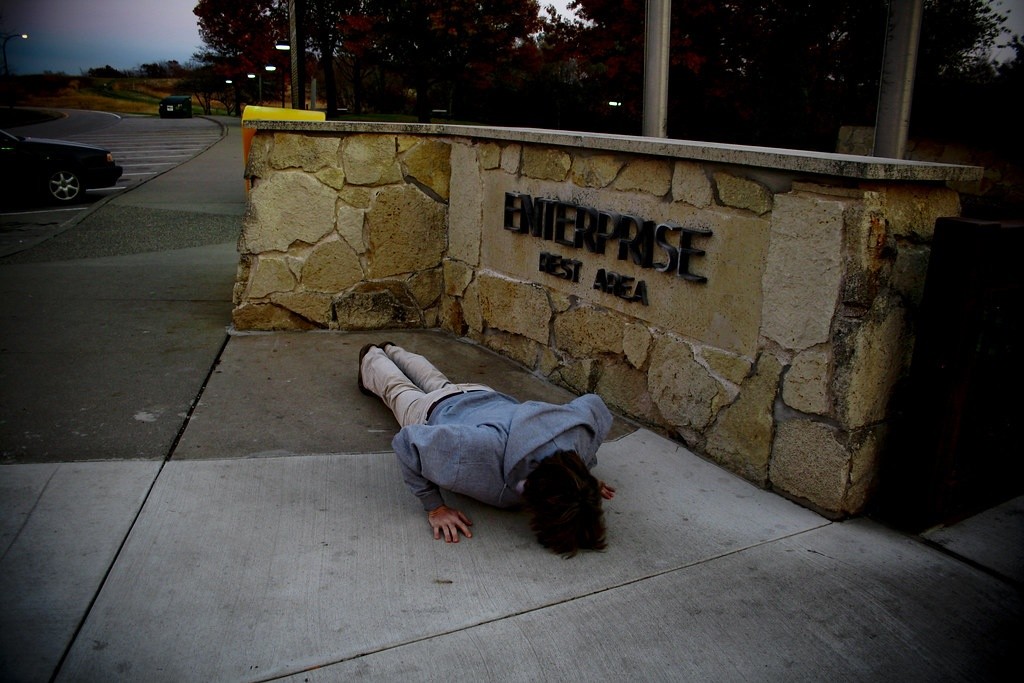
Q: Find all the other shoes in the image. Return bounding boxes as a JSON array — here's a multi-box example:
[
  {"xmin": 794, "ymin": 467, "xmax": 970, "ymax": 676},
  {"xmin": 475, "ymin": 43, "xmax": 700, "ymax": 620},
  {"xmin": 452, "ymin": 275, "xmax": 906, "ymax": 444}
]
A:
[
  {"xmin": 378, "ymin": 341, "xmax": 396, "ymax": 350},
  {"xmin": 357, "ymin": 343, "xmax": 379, "ymax": 397}
]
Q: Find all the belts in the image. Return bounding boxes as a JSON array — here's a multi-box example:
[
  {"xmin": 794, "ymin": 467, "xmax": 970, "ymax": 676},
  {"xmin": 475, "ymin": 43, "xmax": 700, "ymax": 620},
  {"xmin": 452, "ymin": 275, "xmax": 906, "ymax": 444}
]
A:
[{"xmin": 425, "ymin": 390, "xmax": 487, "ymax": 421}]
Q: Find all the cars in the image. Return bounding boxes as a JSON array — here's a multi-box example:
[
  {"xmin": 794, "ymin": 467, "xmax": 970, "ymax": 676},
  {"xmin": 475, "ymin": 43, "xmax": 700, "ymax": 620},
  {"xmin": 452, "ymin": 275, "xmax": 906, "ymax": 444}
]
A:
[{"xmin": 0, "ymin": 130, "xmax": 123, "ymax": 208}]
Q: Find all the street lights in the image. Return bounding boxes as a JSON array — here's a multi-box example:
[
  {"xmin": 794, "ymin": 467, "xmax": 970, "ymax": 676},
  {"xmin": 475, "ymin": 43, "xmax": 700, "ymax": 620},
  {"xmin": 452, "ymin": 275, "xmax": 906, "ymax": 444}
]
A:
[
  {"xmin": 265, "ymin": 60, "xmax": 285, "ymax": 108},
  {"xmin": 247, "ymin": 70, "xmax": 262, "ymax": 106},
  {"xmin": 3, "ymin": 34, "xmax": 28, "ymax": 109},
  {"xmin": 225, "ymin": 78, "xmax": 240, "ymax": 116}
]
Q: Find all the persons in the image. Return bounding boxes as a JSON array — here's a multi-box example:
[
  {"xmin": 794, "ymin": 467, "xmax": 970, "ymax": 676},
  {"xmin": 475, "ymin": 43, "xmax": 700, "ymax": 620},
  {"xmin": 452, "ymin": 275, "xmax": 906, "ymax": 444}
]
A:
[{"xmin": 359, "ymin": 343, "xmax": 616, "ymax": 562}]
